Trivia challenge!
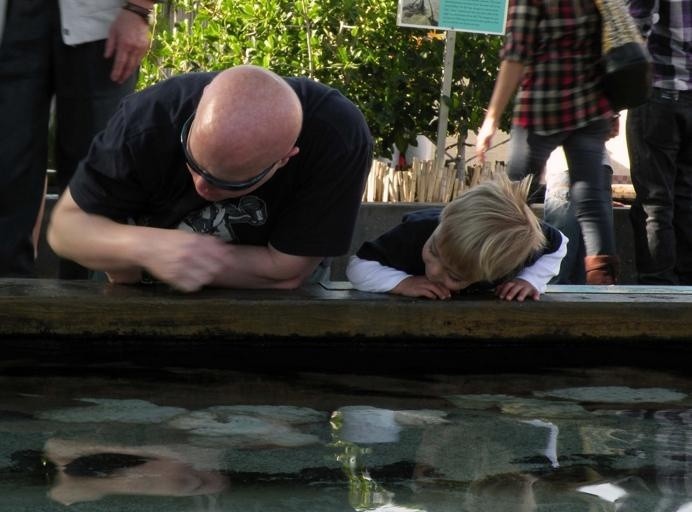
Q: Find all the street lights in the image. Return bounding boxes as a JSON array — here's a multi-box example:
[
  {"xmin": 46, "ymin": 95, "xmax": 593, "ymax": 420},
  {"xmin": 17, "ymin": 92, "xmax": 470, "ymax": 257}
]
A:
[{"xmin": 598, "ymin": 0, "xmax": 652, "ymax": 111}]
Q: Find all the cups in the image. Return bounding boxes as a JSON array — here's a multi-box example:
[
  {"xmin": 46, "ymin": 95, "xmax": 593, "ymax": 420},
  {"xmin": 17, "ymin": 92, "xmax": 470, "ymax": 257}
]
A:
[{"xmin": 179, "ymin": 109, "xmax": 278, "ymax": 191}]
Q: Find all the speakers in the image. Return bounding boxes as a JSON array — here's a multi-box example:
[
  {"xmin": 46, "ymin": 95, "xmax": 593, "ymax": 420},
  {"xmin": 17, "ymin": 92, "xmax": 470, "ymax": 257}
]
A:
[{"xmin": 584, "ymin": 256, "xmax": 615, "ymax": 285}]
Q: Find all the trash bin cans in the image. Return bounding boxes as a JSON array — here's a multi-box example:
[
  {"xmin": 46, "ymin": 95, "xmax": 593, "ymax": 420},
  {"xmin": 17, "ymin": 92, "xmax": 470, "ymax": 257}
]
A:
[
  {"xmin": 2, "ymin": 0, "xmax": 155, "ymax": 282},
  {"xmin": 344, "ymin": 176, "xmax": 570, "ymax": 303},
  {"xmin": 123, "ymin": 0, "xmax": 155, "ymax": 27},
  {"xmin": 624, "ymin": 0, "xmax": 692, "ymax": 286},
  {"xmin": 48, "ymin": 64, "xmax": 373, "ymax": 293}
]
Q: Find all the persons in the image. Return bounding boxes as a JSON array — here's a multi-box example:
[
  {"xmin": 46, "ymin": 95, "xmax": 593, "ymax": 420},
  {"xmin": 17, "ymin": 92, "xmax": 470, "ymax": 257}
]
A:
[{"xmin": 475, "ymin": 0, "xmax": 622, "ymax": 286}]
